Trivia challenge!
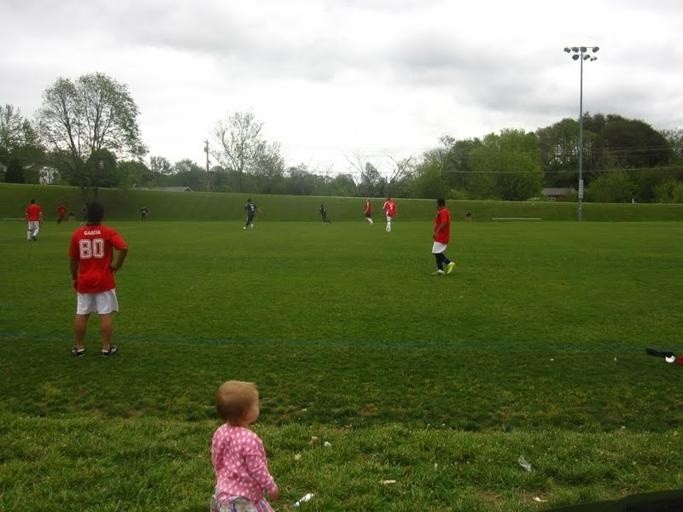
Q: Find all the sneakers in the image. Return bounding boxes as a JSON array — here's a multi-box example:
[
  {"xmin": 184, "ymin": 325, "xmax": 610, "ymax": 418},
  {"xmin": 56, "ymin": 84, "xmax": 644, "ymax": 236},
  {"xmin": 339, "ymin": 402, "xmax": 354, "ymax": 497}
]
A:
[
  {"xmin": 447, "ymin": 262, "xmax": 455, "ymax": 274},
  {"xmin": 432, "ymin": 269, "xmax": 445, "ymax": 275},
  {"xmin": 100, "ymin": 344, "xmax": 118, "ymax": 358},
  {"xmin": 71, "ymin": 345, "xmax": 87, "ymax": 358}
]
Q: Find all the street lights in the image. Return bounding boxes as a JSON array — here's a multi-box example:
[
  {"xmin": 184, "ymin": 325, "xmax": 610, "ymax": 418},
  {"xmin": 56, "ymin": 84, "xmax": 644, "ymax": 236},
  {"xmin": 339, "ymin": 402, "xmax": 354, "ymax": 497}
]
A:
[{"xmin": 563, "ymin": 44, "xmax": 601, "ymax": 222}]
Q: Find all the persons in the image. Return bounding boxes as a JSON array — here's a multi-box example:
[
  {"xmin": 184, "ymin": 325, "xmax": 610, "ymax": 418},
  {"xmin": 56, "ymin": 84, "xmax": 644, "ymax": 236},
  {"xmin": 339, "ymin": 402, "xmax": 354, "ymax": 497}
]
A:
[
  {"xmin": 140, "ymin": 206, "xmax": 148, "ymax": 222},
  {"xmin": 431, "ymin": 198, "xmax": 456, "ymax": 274},
  {"xmin": 242, "ymin": 198, "xmax": 260, "ymax": 230},
  {"xmin": 68, "ymin": 202, "xmax": 128, "ymax": 358},
  {"xmin": 55, "ymin": 202, "xmax": 66, "ymax": 225},
  {"xmin": 320, "ymin": 204, "xmax": 332, "ymax": 223},
  {"xmin": 363, "ymin": 199, "xmax": 374, "ymax": 225},
  {"xmin": 209, "ymin": 380, "xmax": 279, "ymax": 512},
  {"xmin": 382, "ymin": 198, "xmax": 398, "ymax": 232},
  {"xmin": 24, "ymin": 199, "xmax": 43, "ymax": 241}
]
